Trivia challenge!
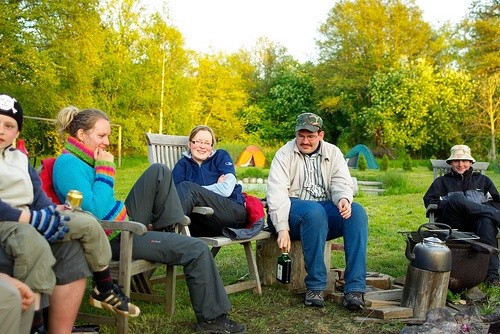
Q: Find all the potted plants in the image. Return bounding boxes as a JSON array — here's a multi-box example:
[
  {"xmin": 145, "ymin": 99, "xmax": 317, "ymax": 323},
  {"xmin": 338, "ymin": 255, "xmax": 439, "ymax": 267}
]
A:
[{"xmin": 236, "ymin": 168, "xmax": 269, "ymax": 184}]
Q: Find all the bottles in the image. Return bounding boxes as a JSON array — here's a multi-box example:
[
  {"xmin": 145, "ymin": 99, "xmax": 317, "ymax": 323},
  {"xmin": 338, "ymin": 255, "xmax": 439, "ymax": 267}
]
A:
[{"xmin": 275, "ymin": 248, "xmax": 291, "ymax": 285}]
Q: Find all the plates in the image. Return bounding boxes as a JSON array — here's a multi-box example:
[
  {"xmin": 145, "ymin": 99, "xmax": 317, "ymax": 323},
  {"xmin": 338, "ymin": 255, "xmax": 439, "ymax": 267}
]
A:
[{"xmin": 427, "ymin": 227, "xmax": 459, "ymax": 232}]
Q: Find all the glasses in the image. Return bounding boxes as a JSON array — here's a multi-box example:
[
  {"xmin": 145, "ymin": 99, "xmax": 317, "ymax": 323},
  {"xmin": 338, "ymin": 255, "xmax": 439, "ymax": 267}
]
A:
[
  {"xmin": 295, "ymin": 134, "xmax": 318, "ymax": 140},
  {"xmin": 189, "ymin": 139, "xmax": 212, "ymax": 147}
]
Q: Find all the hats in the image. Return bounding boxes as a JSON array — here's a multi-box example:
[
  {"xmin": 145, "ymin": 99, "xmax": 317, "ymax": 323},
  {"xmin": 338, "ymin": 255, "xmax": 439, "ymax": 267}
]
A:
[
  {"xmin": 446, "ymin": 145, "xmax": 476, "ymax": 163},
  {"xmin": 0, "ymin": 95, "xmax": 23, "ymax": 131},
  {"xmin": 295, "ymin": 113, "xmax": 322, "ymax": 132}
]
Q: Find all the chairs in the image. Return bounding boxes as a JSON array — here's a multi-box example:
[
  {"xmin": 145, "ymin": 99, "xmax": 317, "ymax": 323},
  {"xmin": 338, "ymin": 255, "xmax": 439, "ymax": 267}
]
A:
[
  {"xmin": 36, "ymin": 158, "xmax": 177, "ymax": 334},
  {"xmin": 426, "ymin": 158, "xmax": 500, "ymax": 257},
  {"xmin": 146, "ymin": 134, "xmax": 271, "ymax": 297}
]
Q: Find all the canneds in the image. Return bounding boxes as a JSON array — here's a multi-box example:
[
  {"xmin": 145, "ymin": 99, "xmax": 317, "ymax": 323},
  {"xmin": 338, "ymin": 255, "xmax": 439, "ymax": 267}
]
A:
[{"xmin": 63, "ymin": 190, "xmax": 83, "ymax": 212}]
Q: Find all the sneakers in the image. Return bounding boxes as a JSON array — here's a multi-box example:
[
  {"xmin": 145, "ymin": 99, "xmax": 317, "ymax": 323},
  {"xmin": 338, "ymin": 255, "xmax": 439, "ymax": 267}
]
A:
[
  {"xmin": 306, "ymin": 288, "xmax": 324, "ymax": 307},
  {"xmin": 196, "ymin": 312, "xmax": 245, "ymax": 334},
  {"xmin": 88, "ymin": 284, "xmax": 140, "ymax": 317},
  {"xmin": 343, "ymin": 292, "xmax": 364, "ymax": 310}
]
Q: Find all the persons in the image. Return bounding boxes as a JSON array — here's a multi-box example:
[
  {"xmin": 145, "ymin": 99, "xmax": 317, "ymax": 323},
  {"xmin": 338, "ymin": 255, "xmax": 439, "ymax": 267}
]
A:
[
  {"xmin": 167, "ymin": 124, "xmax": 248, "ymax": 239},
  {"xmin": 49, "ymin": 106, "xmax": 248, "ymax": 334},
  {"xmin": 0, "ymin": 95, "xmax": 140, "ymax": 334},
  {"xmin": 267, "ymin": 113, "xmax": 368, "ymax": 310},
  {"xmin": 422, "ymin": 144, "xmax": 500, "ymax": 272}
]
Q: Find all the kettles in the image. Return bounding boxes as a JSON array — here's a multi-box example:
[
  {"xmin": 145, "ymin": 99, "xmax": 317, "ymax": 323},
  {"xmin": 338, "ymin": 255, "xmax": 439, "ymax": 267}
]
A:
[{"xmin": 404, "ymin": 222, "xmax": 453, "ymax": 272}]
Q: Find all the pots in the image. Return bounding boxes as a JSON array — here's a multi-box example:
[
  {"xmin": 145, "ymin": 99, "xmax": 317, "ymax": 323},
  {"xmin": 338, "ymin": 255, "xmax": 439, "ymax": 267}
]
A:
[{"xmin": 408, "ymin": 237, "xmax": 490, "ymax": 292}]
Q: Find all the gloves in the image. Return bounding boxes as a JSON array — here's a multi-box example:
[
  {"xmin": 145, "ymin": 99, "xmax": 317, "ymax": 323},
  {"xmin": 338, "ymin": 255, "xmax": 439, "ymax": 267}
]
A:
[{"xmin": 30, "ymin": 203, "xmax": 71, "ymax": 241}]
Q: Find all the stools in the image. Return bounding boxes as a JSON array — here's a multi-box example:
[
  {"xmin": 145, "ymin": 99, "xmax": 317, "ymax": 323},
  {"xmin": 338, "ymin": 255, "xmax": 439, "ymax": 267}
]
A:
[{"xmin": 260, "ymin": 234, "xmax": 332, "ymax": 290}]
